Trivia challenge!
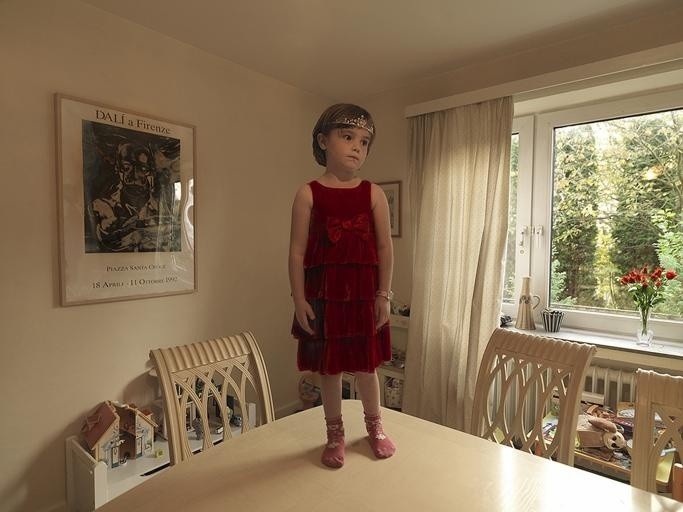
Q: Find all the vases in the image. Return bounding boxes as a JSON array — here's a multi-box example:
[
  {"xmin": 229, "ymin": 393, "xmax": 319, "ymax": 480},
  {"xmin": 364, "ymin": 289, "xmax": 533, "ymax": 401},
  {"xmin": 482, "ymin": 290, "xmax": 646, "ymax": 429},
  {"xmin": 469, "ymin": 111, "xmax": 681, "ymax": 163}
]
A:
[{"xmin": 636, "ymin": 304, "xmax": 654, "ymax": 347}]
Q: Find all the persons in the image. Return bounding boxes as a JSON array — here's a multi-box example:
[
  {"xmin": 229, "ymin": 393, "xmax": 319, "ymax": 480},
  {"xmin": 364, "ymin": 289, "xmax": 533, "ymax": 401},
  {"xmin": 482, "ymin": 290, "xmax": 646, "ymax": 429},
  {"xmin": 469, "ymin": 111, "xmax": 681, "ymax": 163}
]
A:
[{"xmin": 288, "ymin": 102, "xmax": 396, "ymax": 468}]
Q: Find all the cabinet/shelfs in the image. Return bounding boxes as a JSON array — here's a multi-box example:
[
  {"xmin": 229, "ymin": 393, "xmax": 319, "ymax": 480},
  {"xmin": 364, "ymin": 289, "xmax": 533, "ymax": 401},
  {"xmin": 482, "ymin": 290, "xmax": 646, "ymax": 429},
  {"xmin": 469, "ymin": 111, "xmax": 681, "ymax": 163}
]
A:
[
  {"xmin": 300, "ymin": 310, "xmax": 413, "ymax": 413},
  {"xmin": 64, "ymin": 398, "xmax": 258, "ymax": 511}
]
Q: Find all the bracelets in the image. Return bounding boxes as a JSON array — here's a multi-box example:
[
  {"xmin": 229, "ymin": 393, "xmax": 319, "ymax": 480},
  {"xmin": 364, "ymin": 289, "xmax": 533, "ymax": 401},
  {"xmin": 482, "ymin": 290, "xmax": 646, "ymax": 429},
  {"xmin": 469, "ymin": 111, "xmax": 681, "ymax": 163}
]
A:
[{"xmin": 376, "ymin": 289, "xmax": 394, "ymax": 301}]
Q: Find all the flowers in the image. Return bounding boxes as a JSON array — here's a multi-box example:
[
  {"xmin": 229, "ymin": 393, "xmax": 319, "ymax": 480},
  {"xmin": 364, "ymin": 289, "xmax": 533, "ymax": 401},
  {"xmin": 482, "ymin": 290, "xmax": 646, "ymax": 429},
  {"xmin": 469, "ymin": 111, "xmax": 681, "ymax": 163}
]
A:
[{"xmin": 620, "ymin": 264, "xmax": 676, "ymax": 336}]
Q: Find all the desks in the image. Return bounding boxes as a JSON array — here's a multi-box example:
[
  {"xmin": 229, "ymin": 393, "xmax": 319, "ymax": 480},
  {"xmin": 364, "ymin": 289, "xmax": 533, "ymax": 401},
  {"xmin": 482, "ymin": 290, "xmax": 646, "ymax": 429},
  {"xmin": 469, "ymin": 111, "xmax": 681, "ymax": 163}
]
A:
[
  {"xmin": 94, "ymin": 397, "xmax": 682, "ymax": 511},
  {"xmin": 525, "ymin": 405, "xmax": 676, "ymax": 487}
]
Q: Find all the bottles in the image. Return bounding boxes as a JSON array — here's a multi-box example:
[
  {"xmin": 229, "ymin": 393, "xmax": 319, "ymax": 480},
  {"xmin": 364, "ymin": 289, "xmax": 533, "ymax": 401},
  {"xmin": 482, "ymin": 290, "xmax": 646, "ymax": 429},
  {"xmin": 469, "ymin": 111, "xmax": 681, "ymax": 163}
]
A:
[{"xmin": 514, "ymin": 276, "xmax": 541, "ymax": 330}]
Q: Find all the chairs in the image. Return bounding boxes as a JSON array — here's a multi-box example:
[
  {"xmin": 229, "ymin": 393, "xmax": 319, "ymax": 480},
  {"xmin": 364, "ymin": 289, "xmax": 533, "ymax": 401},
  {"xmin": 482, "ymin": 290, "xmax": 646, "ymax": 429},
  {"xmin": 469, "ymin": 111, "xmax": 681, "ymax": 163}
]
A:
[
  {"xmin": 629, "ymin": 367, "xmax": 683, "ymax": 503},
  {"xmin": 147, "ymin": 330, "xmax": 275, "ymax": 467},
  {"xmin": 470, "ymin": 326, "xmax": 598, "ymax": 470}
]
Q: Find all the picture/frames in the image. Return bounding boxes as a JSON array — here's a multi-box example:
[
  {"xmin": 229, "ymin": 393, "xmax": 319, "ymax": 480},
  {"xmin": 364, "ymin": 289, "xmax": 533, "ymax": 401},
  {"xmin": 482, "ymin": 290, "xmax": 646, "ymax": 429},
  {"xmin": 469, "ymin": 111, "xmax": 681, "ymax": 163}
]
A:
[
  {"xmin": 52, "ymin": 91, "xmax": 198, "ymax": 308},
  {"xmin": 373, "ymin": 180, "xmax": 403, "ymax": 239}
]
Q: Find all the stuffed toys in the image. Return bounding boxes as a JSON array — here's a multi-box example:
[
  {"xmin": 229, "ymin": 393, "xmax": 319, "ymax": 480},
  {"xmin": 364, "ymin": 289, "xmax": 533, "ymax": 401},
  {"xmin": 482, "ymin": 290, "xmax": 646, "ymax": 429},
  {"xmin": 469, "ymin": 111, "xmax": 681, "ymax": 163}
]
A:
[{"xmin": 572, "ymin": 417, "xmax": 626, "ymax": 461}]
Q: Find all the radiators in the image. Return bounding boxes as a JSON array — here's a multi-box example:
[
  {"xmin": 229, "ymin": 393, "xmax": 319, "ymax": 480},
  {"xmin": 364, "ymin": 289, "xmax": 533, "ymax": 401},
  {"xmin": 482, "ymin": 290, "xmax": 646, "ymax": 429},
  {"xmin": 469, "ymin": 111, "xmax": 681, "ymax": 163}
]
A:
[{"xmin": 543, "ymin": 364, "xmax": 637, "ymax": 412}]
